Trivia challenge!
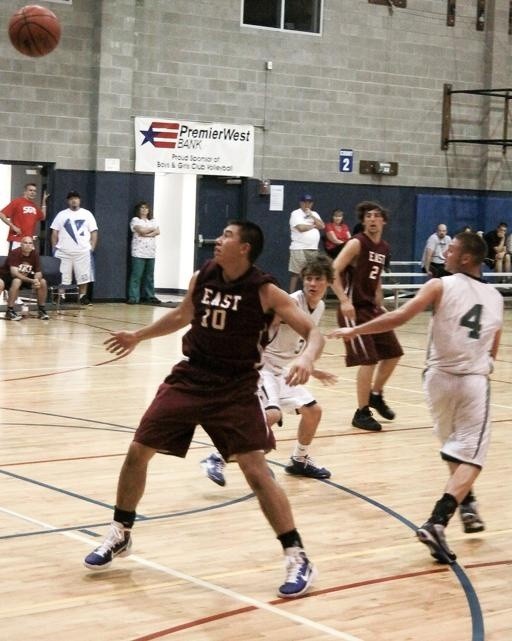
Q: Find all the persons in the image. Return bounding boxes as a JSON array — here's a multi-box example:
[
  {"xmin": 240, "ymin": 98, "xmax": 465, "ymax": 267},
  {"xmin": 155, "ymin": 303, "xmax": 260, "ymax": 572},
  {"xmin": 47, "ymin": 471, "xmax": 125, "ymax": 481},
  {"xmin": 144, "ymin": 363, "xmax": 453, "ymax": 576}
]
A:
[
  {"xmin": 329, "ymin": 200, "xmax": 406, "ymax": 433},
  {"xmin": 128, "ymin": 203, "xmax": 163, "ymax": 305},
  {"xmin": 51, "ymin": 191, "xmax": 98, "ymax": 306},
  {"xmin": 283, "ymin": 196, "xmax": 324, "ymax": 294},
  {"xmin": 197, "ymin": 255, "xmax": 336, "ymax": 486},
  {"xmin": 460, "ymin": 227, "xmax": 472, "ymax": 233},
  {"xmin": 421, "ymin": 224, "xmax": 452, "ymax": 280},
  {"xmin": 325, "ymin": 209, "xmax": 352, "ymax": 262},
  {"xmin": 481, "ymin": 224, "xmax": 511, "ymax": 284},
  {"xmin": 323, "ymin": 232, "xmax": 504, "ymax": 564},
  {"xmin": 506, "ymin": 233, "xmax": 512, "ymax": 273},
  {"xmin": 3, "ymin": 236, "xmax": 49, "ymax": 321},
  {"xmin": 0, "ymin": 182, "xmax": 51, "ymax": 257},
  {"xmin": 83, "ymin": 219, "xmax": 325, "ymax": 598}
]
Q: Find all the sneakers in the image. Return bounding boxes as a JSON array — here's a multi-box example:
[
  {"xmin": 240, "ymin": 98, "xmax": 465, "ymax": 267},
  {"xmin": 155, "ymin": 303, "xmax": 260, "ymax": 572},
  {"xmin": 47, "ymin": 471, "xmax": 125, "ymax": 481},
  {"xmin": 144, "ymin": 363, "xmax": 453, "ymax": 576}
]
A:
[
  {"xmin": 457, "ymin": 493, "xmax": 484, "ymax": 534},
  {"xmin": 144, "ymin": 297, "xmax": 160, "ymax": 304},
  {"xmin": 199, "ymin": 450, "xmax": 227, "ymax": 486},
  {"xmin": 5, "ymin": 306, "xmax": 23, "ymax": 321},
  {"xmin": 284, "ymin": 454, "xmax": 331, "ymax": 480},
  {"xmin": 127, "ymin": 298, "xmax": 138, "ymax": 303},
  {"xmin": 83, "ymin": 518, "xmax": 133, "ymax": 571},
  {"xmin": 351, "ymin": 406, "xmax": 383, "ymax": 433},
  {"xmin": 37, "ymin": 305, "xmax": 50, "ymax": 320},
  {"xmin": 368, "ymin": 389, "xmax": 395, "ymax": 420},
  {"xmin": 276, "ymin": 547, "xmax": 314, "ymax": 598},
  {"xmin": 414, "ymin": 519, "xmax": 458, "ymax": 565},
  {"xmin": 78, "ymin": 293, "xmax": 94, "ymax": 306}
]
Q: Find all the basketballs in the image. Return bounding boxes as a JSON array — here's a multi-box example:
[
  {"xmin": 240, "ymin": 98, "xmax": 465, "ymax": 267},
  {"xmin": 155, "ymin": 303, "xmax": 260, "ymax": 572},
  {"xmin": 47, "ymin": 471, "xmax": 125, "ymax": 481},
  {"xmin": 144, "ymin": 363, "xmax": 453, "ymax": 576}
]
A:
[{"xmin": 6, "ymin": 1, "xmax": 63, "ymax": 60}]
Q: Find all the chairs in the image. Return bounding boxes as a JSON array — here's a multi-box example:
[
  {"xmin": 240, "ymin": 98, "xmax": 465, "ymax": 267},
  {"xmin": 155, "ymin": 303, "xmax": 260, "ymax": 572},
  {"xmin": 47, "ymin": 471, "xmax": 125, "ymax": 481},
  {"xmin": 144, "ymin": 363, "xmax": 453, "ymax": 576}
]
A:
[
  {"xmin": 38, "ymin": 255, "xmax": 80, "ymax": 315},
  {"xmin": 0, "ymin": 256, "xmax": 30, "ymax": 311}
]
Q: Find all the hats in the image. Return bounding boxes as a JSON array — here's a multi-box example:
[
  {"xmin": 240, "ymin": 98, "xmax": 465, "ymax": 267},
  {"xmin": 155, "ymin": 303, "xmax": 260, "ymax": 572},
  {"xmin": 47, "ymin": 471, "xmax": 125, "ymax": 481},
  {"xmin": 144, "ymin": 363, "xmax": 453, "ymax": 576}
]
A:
[{"xmin": 298, "ymin": 192, "xmax": 314, "ymax": 202}]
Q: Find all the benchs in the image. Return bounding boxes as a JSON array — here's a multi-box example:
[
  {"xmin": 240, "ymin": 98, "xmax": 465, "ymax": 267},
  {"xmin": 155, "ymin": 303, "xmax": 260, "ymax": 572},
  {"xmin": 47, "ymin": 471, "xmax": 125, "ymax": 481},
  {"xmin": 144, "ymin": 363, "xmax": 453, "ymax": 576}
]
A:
[{"xmin": 377, "ymin": 260, "xmax": 512, "ymax": 310}]
